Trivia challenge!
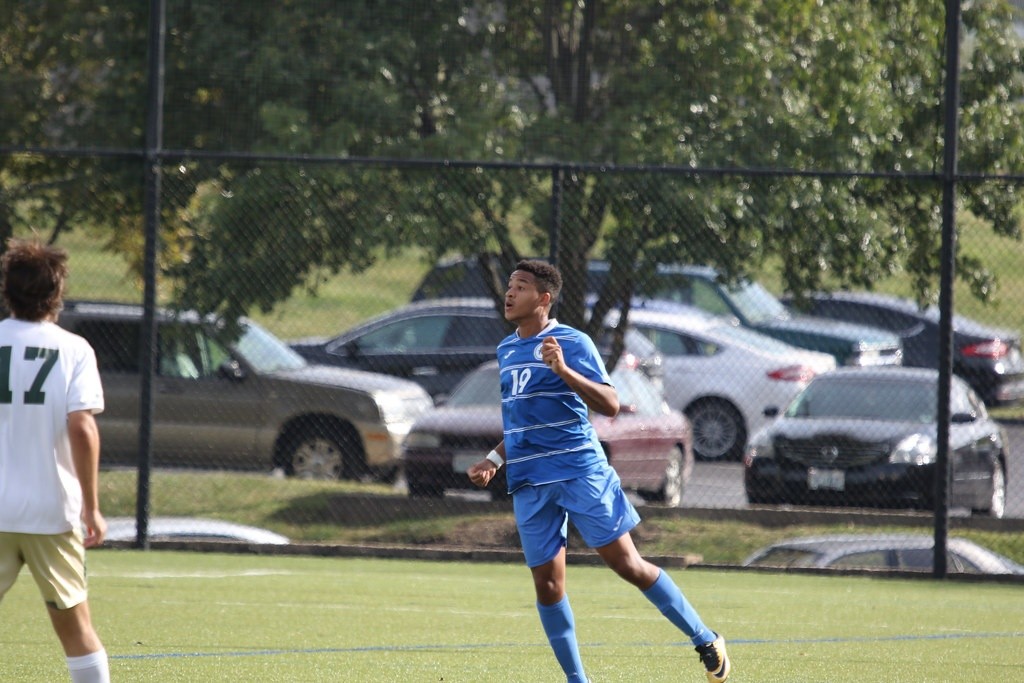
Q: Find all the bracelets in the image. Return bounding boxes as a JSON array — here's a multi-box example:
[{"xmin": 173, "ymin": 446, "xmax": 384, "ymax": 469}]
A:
[{"xmin": 486, "ymin": 449, "xmax": 505, "ymax": 470}]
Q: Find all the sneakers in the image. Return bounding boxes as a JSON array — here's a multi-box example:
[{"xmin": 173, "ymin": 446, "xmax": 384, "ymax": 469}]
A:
[{"xmin": 695, "ymin": 631, "xmax": 730, "ymax": 683}]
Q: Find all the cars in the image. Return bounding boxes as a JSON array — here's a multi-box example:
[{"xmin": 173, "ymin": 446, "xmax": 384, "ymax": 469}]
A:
[
  {"xmin": 583, "ymin": 292, "xmax": 836, "ymax": 463},
  {"xmin": 743, "ymin": 367, "xmax": 1010, "ymax": 523},
  {"xmin": 402, "ymin": 354, "xmax": 694, "ymax": 509},
  {"xmin": 779, "ymin": 292, "xmax": 1023, "ymax": 411},
  {"xmin": 744, "ymin": 532, "xmax": 1024, "ymax": 576}
]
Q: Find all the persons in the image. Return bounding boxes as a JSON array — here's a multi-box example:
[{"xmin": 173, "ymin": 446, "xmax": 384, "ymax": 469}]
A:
[
  {"xmin": 467, "ymin": 261, "xmax": 732, "ymax": 683},
  {"xmin": 0, "ymin": 226, "xmax": 112, "ymax": 683}
]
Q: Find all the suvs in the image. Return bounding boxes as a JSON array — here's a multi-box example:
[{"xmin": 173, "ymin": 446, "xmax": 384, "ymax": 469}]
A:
[
  {"xmin": 55, "ymin": 298, "xmax": 436, "ymax": 487},
  {"xmin": 290, "ymin": 296, "xmax": 667, "ymax": 413},
  {"xmin": 411, "ymin": 252, "xmax": 906, "ymax": 371}
]
